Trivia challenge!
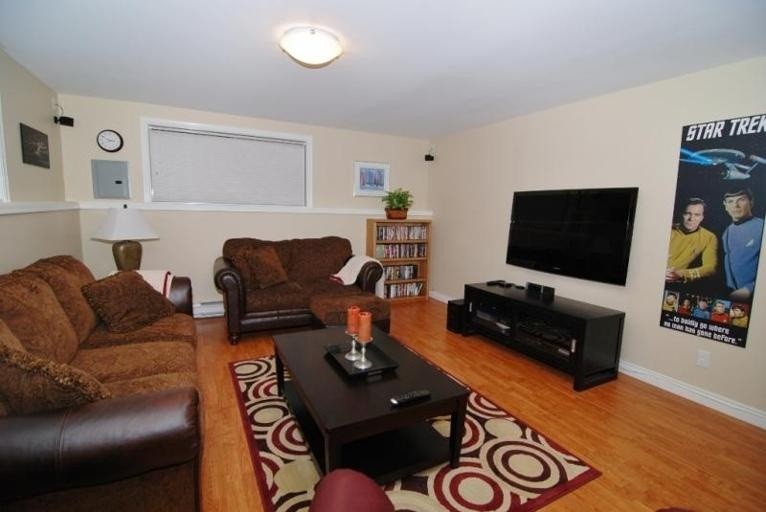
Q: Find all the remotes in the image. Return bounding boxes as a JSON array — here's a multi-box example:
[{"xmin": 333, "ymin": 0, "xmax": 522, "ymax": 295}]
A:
[{"xmin": 389, "ymin": 389, "xmax": 430, "ymax": 406}]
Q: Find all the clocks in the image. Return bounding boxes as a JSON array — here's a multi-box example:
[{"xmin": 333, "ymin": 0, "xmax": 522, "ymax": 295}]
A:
[{"xmin": 97, "ymin": 129, "xmax": 123, "ymax": 152}]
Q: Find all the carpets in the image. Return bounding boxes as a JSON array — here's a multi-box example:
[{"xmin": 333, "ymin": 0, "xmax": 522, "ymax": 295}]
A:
[{"xmin": 226, "ymin": 335, "xmax": 602, "ymax": 512}]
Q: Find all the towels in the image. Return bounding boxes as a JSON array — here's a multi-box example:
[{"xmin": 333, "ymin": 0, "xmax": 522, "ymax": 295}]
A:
[{"xmin": 329, "ymin": 254, "xmax": 385, "ymax": 299}]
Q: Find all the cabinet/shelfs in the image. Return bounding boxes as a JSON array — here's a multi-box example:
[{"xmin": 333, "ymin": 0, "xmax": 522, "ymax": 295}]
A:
[
  {"xmin": 366, "ymin": 217, "xmax": 432, "ymax": 306},
  {"xmin": 447, "ymin": 281, "xmax": 625, "ymax": 392}
]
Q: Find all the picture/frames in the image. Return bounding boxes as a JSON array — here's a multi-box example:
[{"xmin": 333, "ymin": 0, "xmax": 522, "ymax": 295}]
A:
[
  {"xmin": 19, "ymin": 123, "xmax": 50, "ymax": 168},
  {"xmin": 353, "ymin": 162, "xmax": 389, "ymax": 198}
]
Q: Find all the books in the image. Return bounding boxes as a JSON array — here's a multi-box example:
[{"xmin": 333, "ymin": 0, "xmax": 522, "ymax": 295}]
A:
[
  {"xmin": 376, "ymin": 244, "xmax": 426, "ymax": 258},
  {"xmin": 384, "ymin": 282, "xmax": 426, "ymax": 298},
  {"xmin": 376, "ymin": 224, "xmax": 427, "ymax": 240},
  {"xmin": 382, "ymin": 265, "xmax": 418, "ymax": 279}
]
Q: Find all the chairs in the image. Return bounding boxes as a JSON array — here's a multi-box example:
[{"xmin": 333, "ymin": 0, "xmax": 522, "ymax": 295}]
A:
[{"xmin": 308, "ymin": 468, "xmax": 394, "ymax": 511}]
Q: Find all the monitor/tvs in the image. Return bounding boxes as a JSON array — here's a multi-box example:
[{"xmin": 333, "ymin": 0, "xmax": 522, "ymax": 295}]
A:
[{"xmin": 506, "ymin": 187, "xmax": 638, "ymax": 288}]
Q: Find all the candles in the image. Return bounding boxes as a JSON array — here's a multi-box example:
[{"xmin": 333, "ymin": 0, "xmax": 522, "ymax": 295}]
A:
[
  {"xmin": 357, "ymin": 312, "xmax": 372, "ymax": 342},
  {"xmin": 346, "ymin": 305, "xmax": 360, "ymax": 335}
]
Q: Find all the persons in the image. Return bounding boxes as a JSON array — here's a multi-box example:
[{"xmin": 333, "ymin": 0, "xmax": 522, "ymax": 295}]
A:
[
  {"xmin": 730, "ymin": 303, "xmax": 748, "ymax": 328},
  {"xmin": 664, "ymin": 197, "xmax": 718, "ymax": 286},
  {"xmin": 663, "ymin": 292, "xmax": 677, "ymax": 311},
  {"xmin": 722, "ymin": 183, "xmax": 765, "ymax": 299},
  {"xmin": 678, "ymin": 299, "xmax": 692, "ymax": 316},
  {"xmin": 710, "ymin": 301, "xmax": 729, "ymax": 325},
  {"xmin": 692, "ymin": 299, "xmax": 710, "ymax": 319}
]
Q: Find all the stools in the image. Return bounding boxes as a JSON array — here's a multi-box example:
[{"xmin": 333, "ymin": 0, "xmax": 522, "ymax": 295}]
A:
[{"xmin": 310, "ymin": 294, "xmax": 391, "ymax": 333}]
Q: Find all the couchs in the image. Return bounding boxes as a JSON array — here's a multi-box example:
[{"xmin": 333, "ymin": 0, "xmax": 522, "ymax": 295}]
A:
[
  {"xmin": 1, "ymin": 255, "xmax": 203, "ymax": 512},
  {"xmin": 213, "ymin": 236, "xmax": 383, "ymax": 345}
]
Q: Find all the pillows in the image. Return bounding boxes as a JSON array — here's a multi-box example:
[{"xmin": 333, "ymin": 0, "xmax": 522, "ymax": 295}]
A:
[
  {"xmin": 80, "ymin": 270, "xmax": 176, "ymax": 334},
  {"xmin": 231, "ymin": 245, "xmax": 259, "ymax": 293},
  {"xmin": 246, "ymin": 245, "xmax": 288, "ymax": 290},
  {"xmin": 0, "ymin": 341, "xmax": 113, "ymax": 418}
]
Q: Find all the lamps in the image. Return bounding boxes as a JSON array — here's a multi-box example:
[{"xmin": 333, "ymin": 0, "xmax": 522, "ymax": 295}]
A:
[
  {"xmin": 89, "ymin": 201, "xmax": 160, "ymax": 274},
  {"xmin": 276, "ymin": 23, "xmax": 345, "ymax": 67}
]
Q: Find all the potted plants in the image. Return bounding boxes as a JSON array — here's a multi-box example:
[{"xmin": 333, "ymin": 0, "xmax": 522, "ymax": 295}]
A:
[{"xmin": 381, "ymin": 187, "xmax": 415, "ymax": 220}]
